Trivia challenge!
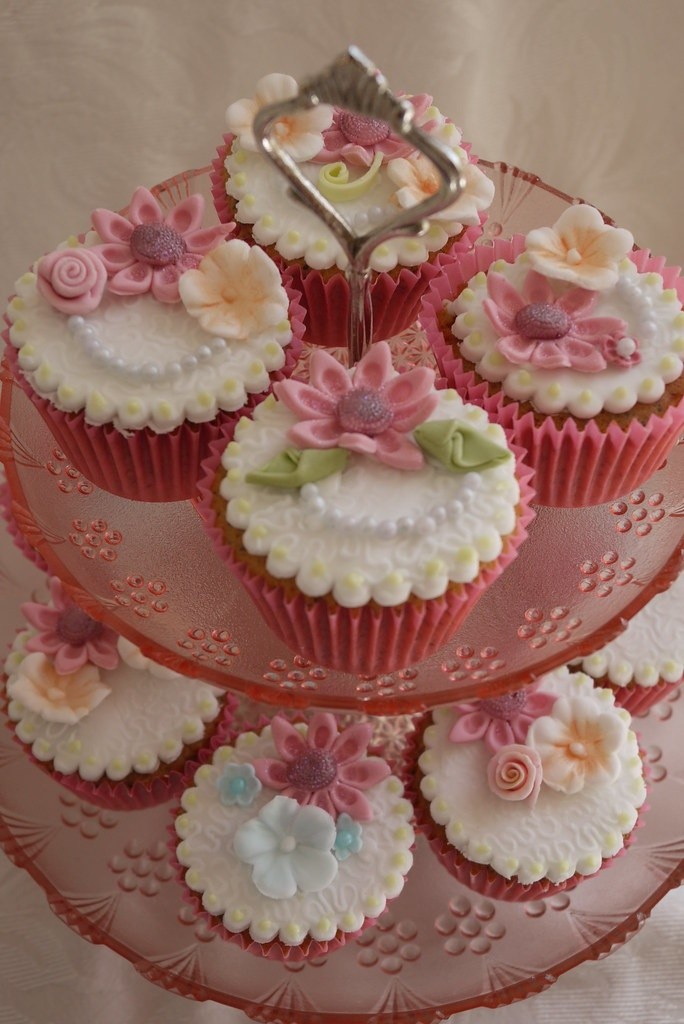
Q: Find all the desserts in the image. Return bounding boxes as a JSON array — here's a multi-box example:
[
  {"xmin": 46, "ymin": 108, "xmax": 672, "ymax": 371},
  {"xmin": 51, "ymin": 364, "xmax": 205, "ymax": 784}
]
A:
[
  {"xmin": 0, "ymin": 564, "xmax": 684, "ymax": 961},
  {"xmin": 0, "ymin": 71, "xmax": 684, "ymax": 677}
]
